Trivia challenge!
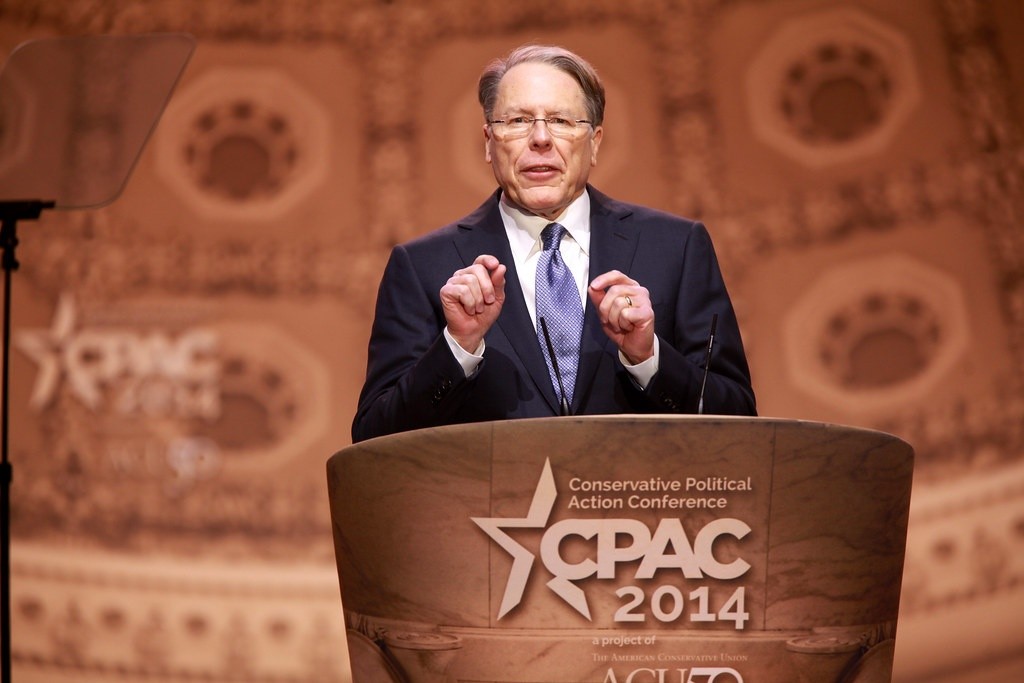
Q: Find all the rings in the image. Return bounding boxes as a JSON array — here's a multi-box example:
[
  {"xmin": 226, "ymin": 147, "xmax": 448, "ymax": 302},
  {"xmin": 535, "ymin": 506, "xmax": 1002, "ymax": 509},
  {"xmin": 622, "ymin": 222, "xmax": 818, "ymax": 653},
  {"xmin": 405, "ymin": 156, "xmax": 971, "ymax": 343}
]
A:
[{"xmin": 625, "ymin": 296, "xmax": 631, "ymax": 305}]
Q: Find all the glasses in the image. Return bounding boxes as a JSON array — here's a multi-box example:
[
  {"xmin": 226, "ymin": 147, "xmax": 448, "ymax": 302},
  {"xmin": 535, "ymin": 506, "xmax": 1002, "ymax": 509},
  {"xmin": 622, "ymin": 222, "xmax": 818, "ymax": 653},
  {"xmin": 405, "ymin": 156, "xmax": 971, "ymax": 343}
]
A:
[{"xmin": 487, "ymin": 113, "xmax": 592, "ymax": 138}]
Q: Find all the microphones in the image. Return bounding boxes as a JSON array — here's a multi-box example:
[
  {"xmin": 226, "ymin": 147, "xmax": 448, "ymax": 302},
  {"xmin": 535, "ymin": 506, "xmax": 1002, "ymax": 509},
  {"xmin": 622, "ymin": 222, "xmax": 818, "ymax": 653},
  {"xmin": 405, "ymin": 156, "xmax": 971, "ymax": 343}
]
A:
[
  {"xmin": 540, "ymin": 317, "xmax": 571, "ymax": 416},
  {"xmin": 699, "ymin": 313, "xmax": 718, "ymax": 415}
]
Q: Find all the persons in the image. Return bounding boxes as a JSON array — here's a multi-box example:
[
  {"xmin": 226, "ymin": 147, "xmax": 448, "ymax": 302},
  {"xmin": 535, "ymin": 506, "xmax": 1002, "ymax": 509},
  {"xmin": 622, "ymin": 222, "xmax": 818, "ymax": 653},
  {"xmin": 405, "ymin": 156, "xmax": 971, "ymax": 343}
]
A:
[{"xmin": 352, "ymin": 45, "xmax": 758, "ymax": 443}]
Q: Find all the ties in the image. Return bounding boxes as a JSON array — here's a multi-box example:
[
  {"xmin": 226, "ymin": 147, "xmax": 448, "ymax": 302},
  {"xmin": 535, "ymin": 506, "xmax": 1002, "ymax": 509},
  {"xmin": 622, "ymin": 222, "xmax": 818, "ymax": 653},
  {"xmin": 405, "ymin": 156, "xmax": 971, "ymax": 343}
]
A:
[{"xmin": 532, "ymin": 223, "xmax": 587, "ymax": 408}]
